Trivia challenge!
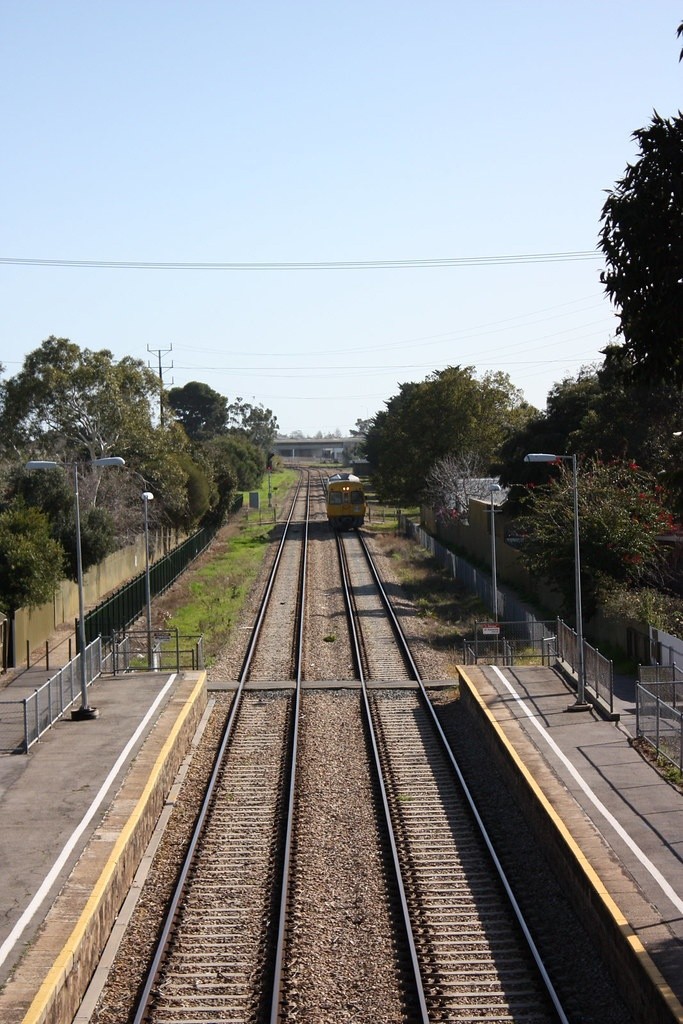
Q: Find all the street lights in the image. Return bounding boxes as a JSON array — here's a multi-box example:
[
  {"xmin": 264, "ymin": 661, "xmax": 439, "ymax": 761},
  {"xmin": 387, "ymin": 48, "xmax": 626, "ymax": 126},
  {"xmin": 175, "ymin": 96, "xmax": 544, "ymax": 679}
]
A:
[
  {"xmin": 27, "ymin": 456, "xmax": 127, "ymax": 722},
  {"xmin": 524, "ymin": 453, "xmax": 595, "ymax": 711},
  {"xmin": 141, "ymin": 492, "xmax": 159, "ymax": 671},
  {"xmin": 481, "ymin": 484, "xmax": 500, "ymax": 664}
]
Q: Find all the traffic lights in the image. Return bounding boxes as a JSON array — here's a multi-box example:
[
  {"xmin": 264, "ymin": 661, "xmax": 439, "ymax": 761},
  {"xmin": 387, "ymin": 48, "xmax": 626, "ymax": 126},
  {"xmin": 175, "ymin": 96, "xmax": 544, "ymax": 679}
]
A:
[{"xmin": 267, "ymin": 452, "xmax": 274, "ymax": 470}]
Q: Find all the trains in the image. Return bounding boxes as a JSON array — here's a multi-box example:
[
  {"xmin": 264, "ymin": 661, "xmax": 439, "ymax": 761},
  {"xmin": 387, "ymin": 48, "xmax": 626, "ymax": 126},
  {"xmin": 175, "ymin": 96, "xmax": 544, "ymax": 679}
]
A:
[{"xmin": 325, "ymin": 472, "xmax": 367, "ymax": 531}]
